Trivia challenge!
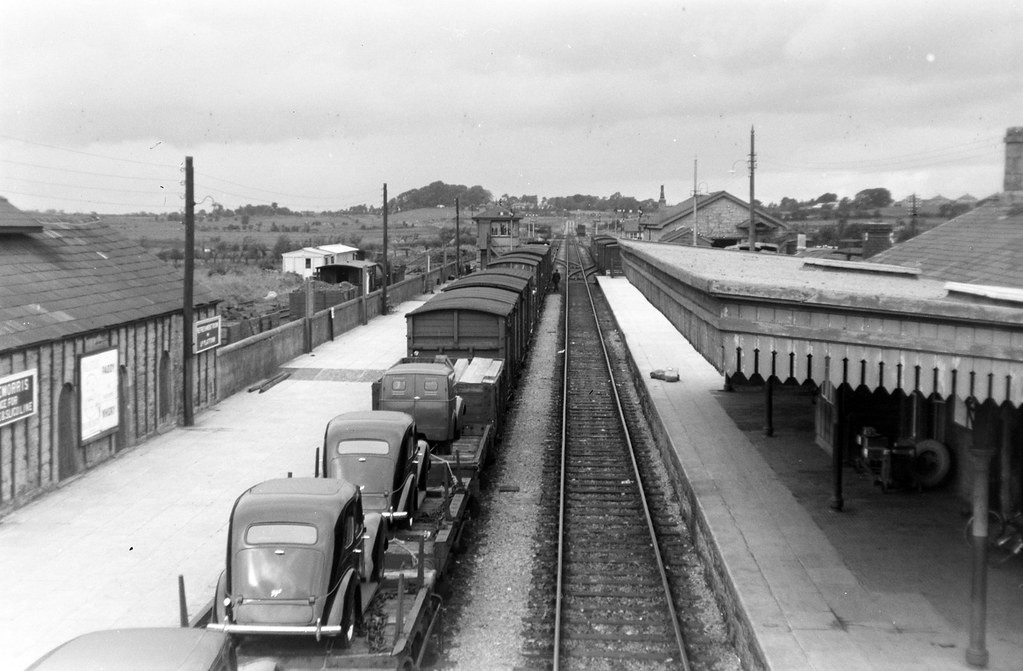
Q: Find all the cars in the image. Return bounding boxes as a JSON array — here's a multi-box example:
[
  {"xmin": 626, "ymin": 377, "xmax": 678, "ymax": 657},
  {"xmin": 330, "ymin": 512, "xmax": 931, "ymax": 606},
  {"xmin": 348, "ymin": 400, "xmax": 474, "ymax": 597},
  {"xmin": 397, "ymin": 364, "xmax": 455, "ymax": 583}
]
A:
[
  {"xmin": 203, "ymin": 472, "xmax": 386, "ymax": 650},
  {"xmin": 314, "ymin": 410, "xmax": 431, "ymax": 526},
  {"xmin": 377, "ymin": 361, "xmax": 467, "ymax": 445},
  {"xmin": 25, "ymin": 626, "xmax": 241, "ymax": 671}
]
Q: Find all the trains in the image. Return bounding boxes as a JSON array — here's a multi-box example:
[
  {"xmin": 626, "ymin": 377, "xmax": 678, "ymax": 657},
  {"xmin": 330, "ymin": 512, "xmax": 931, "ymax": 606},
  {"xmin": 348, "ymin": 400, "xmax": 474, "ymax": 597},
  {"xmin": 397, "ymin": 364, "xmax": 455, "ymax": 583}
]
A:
[{"xmin": 137, "ymin": 243, "xmax": 552, "ymax": 671}]
